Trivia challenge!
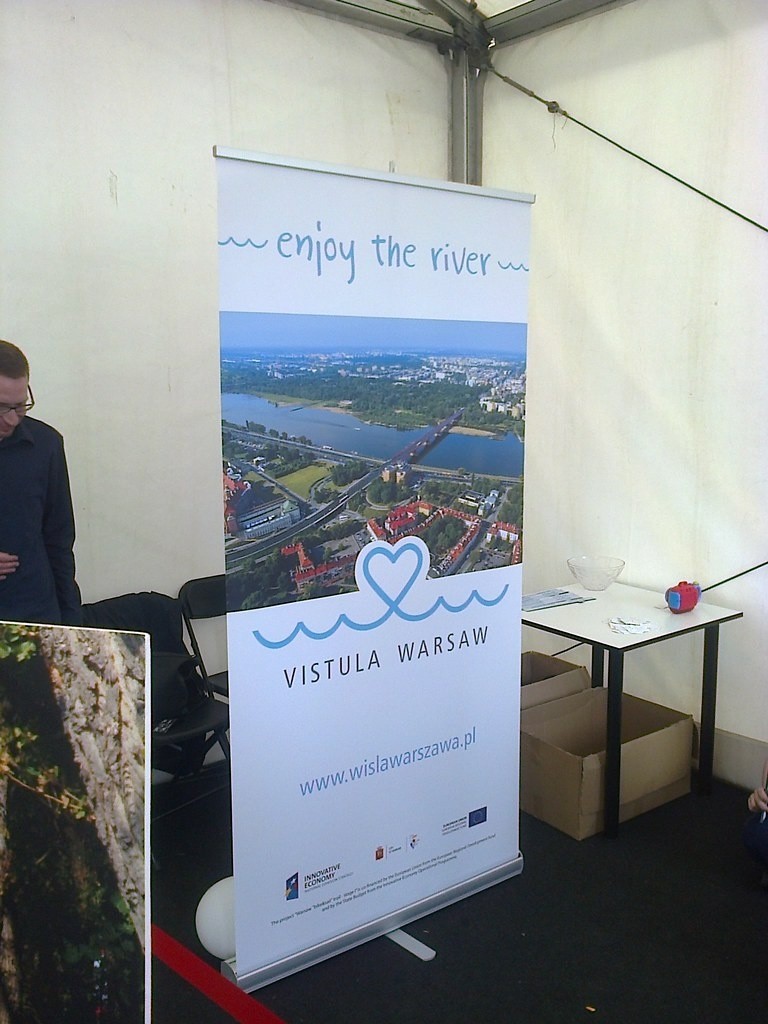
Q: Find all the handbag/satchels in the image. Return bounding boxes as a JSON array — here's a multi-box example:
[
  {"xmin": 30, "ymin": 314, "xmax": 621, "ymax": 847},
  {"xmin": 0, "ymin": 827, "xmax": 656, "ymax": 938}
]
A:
[{"xmin": 151, "ymin": 652, "xmax": 207, "ymax": 718}]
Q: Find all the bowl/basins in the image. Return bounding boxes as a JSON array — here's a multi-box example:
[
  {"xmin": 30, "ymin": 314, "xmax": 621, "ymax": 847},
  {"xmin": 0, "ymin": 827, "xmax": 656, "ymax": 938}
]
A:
[{"xmin": 567, "ymin": 555, "xmax": 626, "ymax": 591}]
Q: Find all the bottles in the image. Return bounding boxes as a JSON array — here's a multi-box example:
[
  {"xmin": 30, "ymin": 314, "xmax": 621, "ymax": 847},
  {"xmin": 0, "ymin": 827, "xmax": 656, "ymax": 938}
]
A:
[{"xmin": 667, "ymin": 579, "xmax": 703, "ymax": 615}]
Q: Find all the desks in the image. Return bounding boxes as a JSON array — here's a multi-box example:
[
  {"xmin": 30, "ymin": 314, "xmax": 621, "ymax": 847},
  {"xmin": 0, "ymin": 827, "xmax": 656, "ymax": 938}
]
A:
[{"xmin": 521, "ymin": 582, "xmax": 743, "ymax": 837}]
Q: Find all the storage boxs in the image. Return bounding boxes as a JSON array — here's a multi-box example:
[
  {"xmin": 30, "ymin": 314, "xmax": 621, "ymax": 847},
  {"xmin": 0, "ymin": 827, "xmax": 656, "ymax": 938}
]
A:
[
  {"xmin": 520, "ymin": 686, "xmax": 700, "ymax": 841},
  {"xmin": 521, "ymin": 650, "xmax": 590, "ymax": 711}
]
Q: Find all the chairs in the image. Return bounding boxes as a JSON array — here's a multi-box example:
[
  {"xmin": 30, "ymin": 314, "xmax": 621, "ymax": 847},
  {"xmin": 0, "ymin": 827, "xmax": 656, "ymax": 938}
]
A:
[
  {"xmin": 82, "ymin": 592, "xmax": 232, "ymax": 824},
  {"xmin": 179, "ymin": 574, "xmax": 232, "ymax": 777}
]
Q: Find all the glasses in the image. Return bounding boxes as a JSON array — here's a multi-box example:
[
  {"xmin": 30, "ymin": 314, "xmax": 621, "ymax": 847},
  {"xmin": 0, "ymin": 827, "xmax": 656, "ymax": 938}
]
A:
[{"xmin": 0, "ymin": 385, "xmax": 34, "ymax": 416}]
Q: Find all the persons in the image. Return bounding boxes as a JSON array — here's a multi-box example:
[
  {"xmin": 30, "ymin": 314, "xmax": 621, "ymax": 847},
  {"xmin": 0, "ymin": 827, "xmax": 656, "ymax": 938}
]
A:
[
  {"xmin": 0, "ymin": 340, "xmax": 85, "ymax": 624},
  {"xmin": 747, "ymin": 758, "xmax": 768, "ymax": 813}
]
